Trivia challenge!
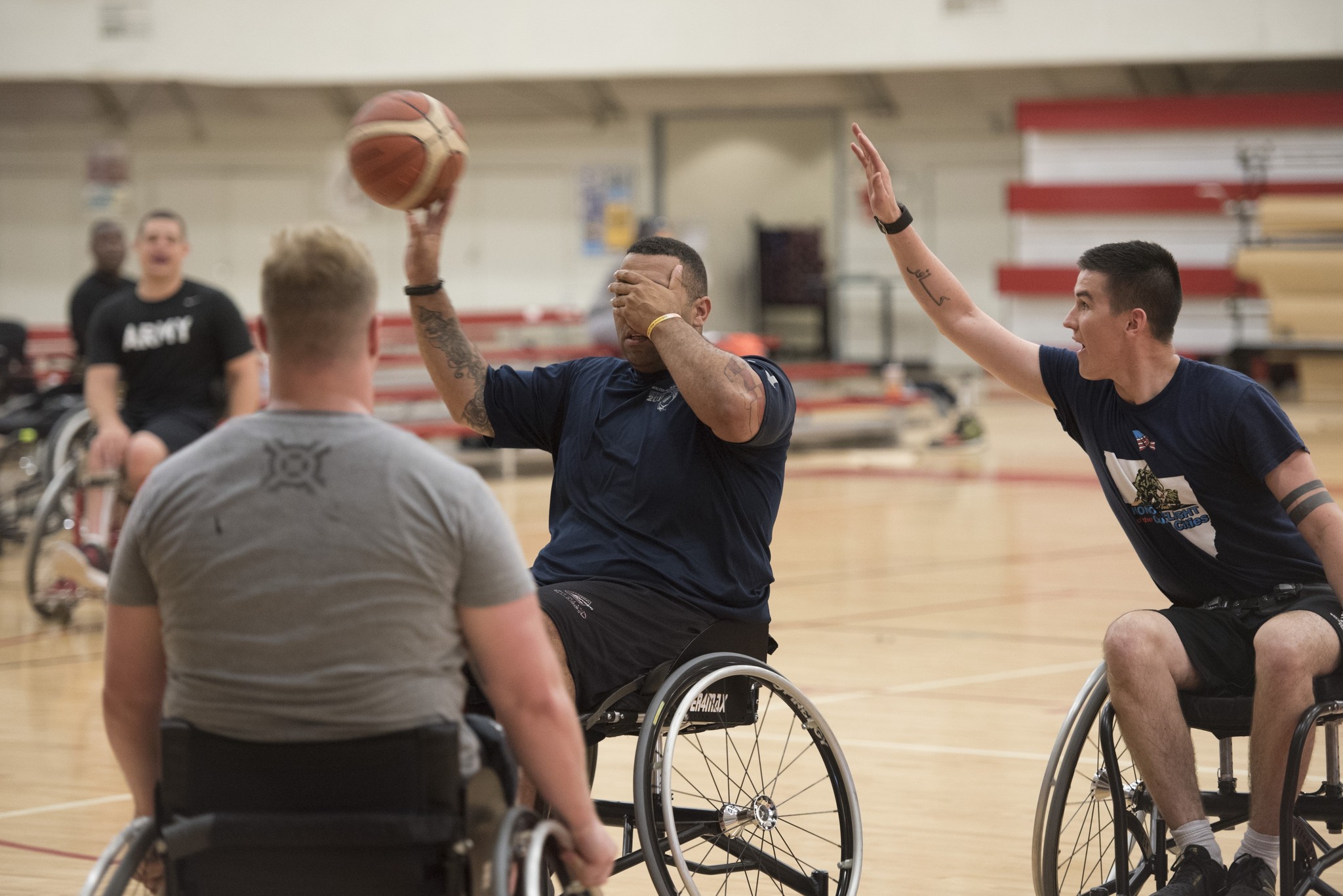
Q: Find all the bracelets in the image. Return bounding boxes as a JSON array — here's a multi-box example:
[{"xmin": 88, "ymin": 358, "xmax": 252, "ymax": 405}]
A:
[
  {"xmin": 646, "ymin": 313, "xmax": 681, "ymax": 341},
  {"xmin": 405, "ymin": 278, "xmax": 442, "ymax": 297}
]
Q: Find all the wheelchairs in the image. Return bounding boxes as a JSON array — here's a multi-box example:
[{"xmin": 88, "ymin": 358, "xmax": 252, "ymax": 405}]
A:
[
  {"xmin": 583, "ymin": 651, "xmax": 865, "ymax": 896},
  {"xmin": 1035, "ymin": 623, "xmax": 1343, "ymax": 896},
  {"xmin": 79, "ymin": 715, "xmax": 581, "ymax": 896},
  {"xmin": 0, "ymin": 380, "xmax": 120, "ymax": 621}
]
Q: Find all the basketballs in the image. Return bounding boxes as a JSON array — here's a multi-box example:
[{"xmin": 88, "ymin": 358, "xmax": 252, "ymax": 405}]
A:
[{"xmin": 345, "ymin": 90, "xmax": 468, "ymax": 211}]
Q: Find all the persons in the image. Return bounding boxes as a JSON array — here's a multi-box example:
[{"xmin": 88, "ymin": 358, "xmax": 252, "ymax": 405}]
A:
[
  {"xmin": 403, "ymin": 185, "xmax": 798, "ymax": 896},
  {"xmin": 0, "ymin": 210, "xmax": 269, "ymax": 607},
  {"xmin": 111, "ymin": 233, "xmax": 616, "ymax": 896},
  {"xmin": 852, "ymin": 124, "xmax": 1343, "ymax": 896}
]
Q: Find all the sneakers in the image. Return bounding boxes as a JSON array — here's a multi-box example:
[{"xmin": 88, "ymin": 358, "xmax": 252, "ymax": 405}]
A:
[
  {"xmin": 1222, "ymin": 852, "xmax": 1277, "ymax": 896},
  {"xmin": 1149, "ymin": 844, "xmax": 1229, "ymax": 896},
  {"xmin": 47, "ymin": 537, "xmax": 110, "ymax": 592}
]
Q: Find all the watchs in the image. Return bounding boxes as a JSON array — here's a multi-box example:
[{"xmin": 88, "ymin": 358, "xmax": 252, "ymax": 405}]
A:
[{"xmin": 873, "ymin": 199, "xmax": 916, "ymax": 233}]
